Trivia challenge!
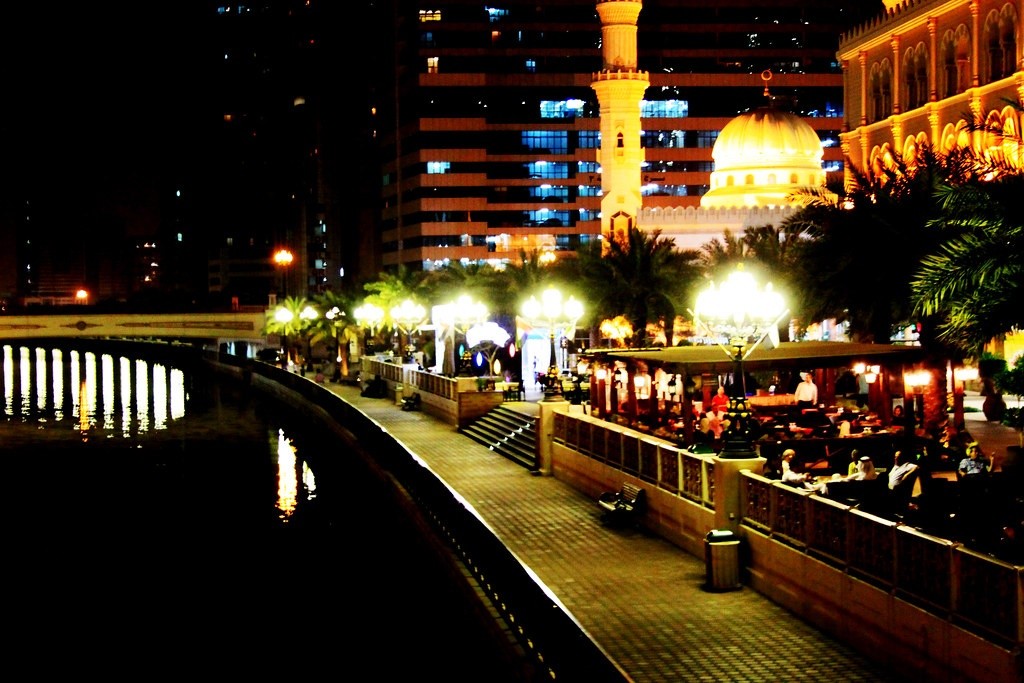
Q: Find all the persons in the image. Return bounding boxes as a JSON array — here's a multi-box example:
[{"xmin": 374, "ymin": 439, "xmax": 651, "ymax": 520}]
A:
[
  {"xmin": 782, "ymin": 449, "xmax": 809, "ymax": 483},
  {"xmin": 957, "ymin": 446, "xmax": 996, "ymax": 477},
  {"xmin": 316, "ymin": 368, "xmax": 325, "ymax": 384},
  {"xmin": 711, "ymin": 386, "xmax": 730, "ymax": 416},
  {"xmin": 794, "ymin": 373, "xmax": 819, "ymax": 408},
  {"xmin": 848, "ymin": 449, "xmax": 877, "ymax": 481},
  {"xmin": 424, "ymin": 335, "xmax": 435, "ymax": 363},
  {"xmin": 888, "ymin": 450, "xmax": 918, "ymax": 490}
]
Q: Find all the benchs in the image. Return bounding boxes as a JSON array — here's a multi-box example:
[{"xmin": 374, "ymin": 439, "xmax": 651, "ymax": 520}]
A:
[
  {"xmin": 401, "ymin": 391, "xmax": 423, "ymax": 410},
  {"xmin": 597, "ymin": 481, "xmax": 645, "ymax": 531}
]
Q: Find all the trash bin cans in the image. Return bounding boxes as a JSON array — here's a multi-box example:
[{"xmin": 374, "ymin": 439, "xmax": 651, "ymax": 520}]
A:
[
  {"xmin": 702, "ymin": 529, "xmax": 742, "ymax": 592},
  {"xmin": 393, "ymin": 383, "xmax": 404, "ymax": 405}
]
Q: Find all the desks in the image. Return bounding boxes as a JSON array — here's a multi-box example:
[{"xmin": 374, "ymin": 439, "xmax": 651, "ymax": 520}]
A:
[{"xmin": 759, "ymin": 407, "xmax": 898, "ymax": 474}]
